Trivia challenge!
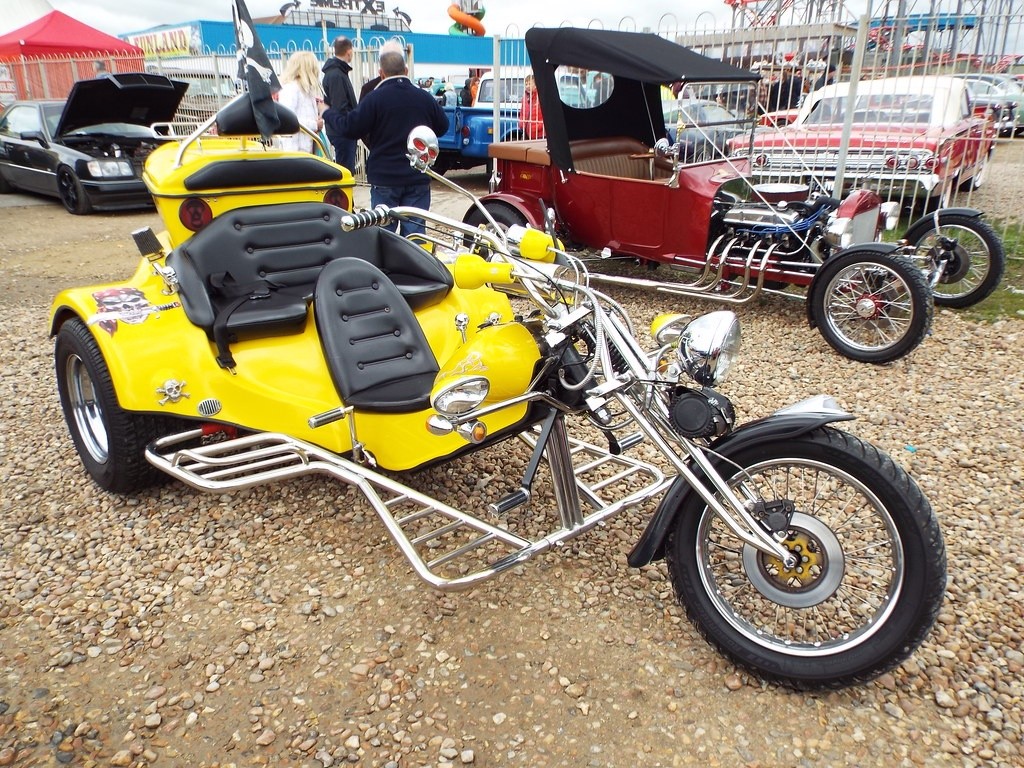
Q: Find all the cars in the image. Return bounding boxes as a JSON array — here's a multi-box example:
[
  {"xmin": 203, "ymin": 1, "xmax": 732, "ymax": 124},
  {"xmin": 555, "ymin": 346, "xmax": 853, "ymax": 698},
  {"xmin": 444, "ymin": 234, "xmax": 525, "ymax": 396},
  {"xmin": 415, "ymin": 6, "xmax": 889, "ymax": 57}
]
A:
[
  {"xmin": 950, "ymin": 70, "xmax": 1024, "ymax": 138},
  {"xmin": 728, "ymin": 73, "xmax": 998, "ymax": 210},
  {"xmin": 0, "ymin": 72, "xmax": 189, "ymax": 214},
  {"xmin": 659, "ymin": 98, "xmax": 777, "ymax": 170}
]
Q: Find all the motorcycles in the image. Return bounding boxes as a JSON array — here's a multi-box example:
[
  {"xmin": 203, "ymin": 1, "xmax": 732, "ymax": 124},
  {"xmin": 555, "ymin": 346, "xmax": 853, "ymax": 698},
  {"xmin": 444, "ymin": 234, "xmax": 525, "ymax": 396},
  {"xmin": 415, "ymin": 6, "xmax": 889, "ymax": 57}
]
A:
[
  {"xmin": 468, "ymin": 20, "xmax": 1005, "ymax": 367},
  {"xmin": 46, "ymin": 1, "xmax": 949, "ymax": 690}
]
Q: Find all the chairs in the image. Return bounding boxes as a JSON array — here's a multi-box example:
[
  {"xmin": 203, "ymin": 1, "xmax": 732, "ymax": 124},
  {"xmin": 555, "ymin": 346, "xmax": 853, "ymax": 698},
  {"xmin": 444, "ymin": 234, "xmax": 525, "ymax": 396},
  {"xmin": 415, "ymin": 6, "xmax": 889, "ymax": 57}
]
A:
[
  {"xmin": 169, "ymin": 197, "xmax": 455, "ymax": 337},
  {"xmin": 563, "ymin": 134, "xmax": 684, "ymax": 186}
]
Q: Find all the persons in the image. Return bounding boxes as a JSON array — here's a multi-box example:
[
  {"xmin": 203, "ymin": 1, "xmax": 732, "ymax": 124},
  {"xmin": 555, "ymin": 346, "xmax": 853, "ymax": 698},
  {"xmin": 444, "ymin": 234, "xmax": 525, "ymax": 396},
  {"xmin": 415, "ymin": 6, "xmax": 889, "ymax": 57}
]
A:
[
  {"xmin": 353, "ymin": 38, "xmax": 426, "ymax": 185},
  {"xmin": 318, "ymin": 48, "xmax": 450, "ymax": 249},
  {"xmin": 517, "ymin": 72, "xmax": 553, "ymax": 143},
  {"xmin": 321, "ymin": 35, "xmax": 363, "ymax": 215},
  {"xmin": 276, "ymin": 47, "xmax": 328, "ymax": 170},
  {"xmin": 459, "ymin": 71, "xmax": 485, "ymax": 111},
  {"xmin": 659, "ymin": 48, "xmax": 867, "ymax": 142}
]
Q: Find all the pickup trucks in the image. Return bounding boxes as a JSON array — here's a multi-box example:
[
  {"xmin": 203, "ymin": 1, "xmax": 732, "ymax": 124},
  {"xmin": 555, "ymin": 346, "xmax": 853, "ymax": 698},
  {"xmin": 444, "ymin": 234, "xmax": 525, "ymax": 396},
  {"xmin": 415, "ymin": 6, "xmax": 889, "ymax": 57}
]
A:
[{"xmin": 425, "ymin": 68, "xmax": 595, "ymax": 185}]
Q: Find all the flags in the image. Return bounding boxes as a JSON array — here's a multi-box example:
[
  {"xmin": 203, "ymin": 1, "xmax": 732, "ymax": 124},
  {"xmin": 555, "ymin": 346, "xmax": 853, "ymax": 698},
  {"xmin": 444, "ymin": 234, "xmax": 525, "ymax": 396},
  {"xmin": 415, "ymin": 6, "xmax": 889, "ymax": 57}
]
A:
[{"xmin": 226, "ymin": 0, "xmax": 284, "ymax": 146}]
